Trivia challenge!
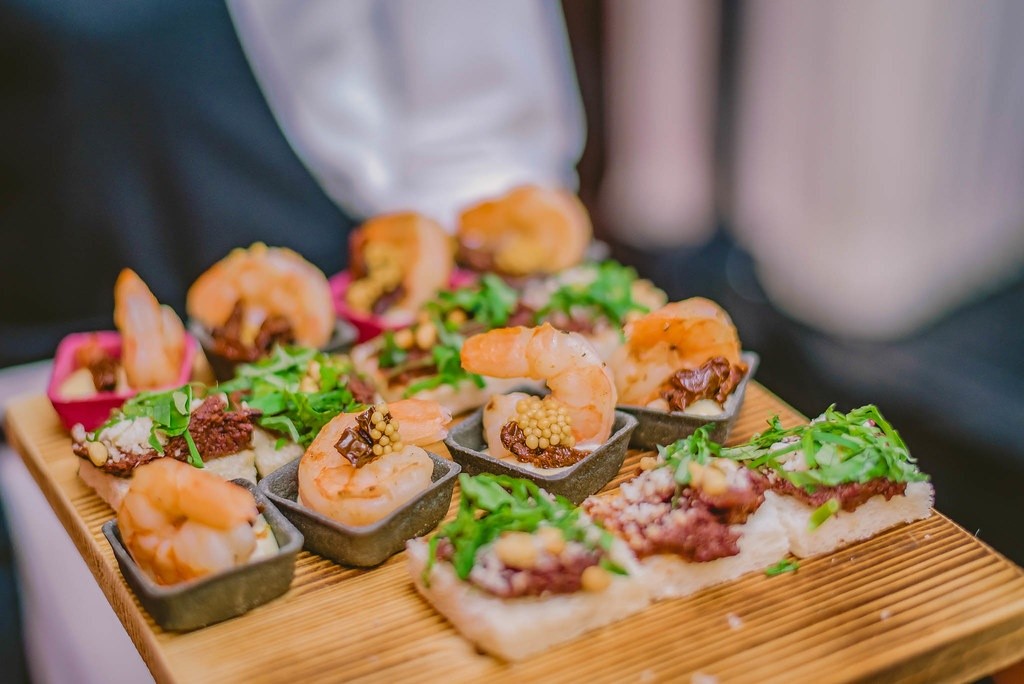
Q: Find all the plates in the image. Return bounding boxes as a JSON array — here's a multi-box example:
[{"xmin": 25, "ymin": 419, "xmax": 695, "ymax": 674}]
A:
[
  {"xmin": 259, "ymin": 443, "xmax": 460, "ymax": 569},
  {"xmin": 461, "ymin": 237, "xmax": 611, "ymax": 283},
  {"xmin": 325, "ymin": 257, "xmax": 478, "ymax": 346},
  {"xmin": 613, "ymin": 352, "xmax": 764, "ymax": 458},
  {"xmin": 443, "ymin": 382, "xmax": 640, "ymax": 503},
  {"xmin": 183, "ymin": 314, "xmax": 357, "ymax": 380},
  {"xmin": 46, "ymin": 330, "xmax": 197, "ymax": 436},
  {"xmin": 102, "ymin": 475, "xmax": 305, "ymax": 635}
]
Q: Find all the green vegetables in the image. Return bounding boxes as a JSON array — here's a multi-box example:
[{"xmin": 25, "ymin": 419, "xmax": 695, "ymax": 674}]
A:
[{"xmin": 118, "ymin": 258, "xmax": 934, "ymax": 582}]
